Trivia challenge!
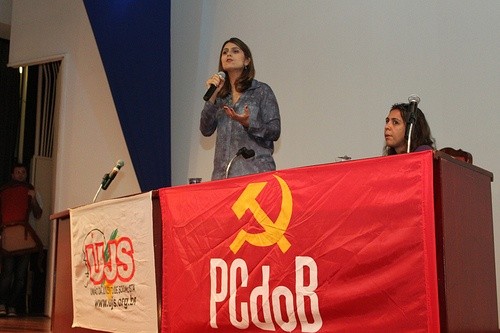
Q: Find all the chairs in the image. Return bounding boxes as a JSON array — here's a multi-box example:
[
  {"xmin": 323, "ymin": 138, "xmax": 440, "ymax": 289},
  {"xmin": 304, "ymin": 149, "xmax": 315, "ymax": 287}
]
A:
[{"xmin": 0, "ymin": 183, "xmax": 44, "ymax": 273}]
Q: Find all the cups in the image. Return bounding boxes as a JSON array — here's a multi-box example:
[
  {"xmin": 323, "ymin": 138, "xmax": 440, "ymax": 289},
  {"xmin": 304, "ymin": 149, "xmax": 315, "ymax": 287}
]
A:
[
  {"xmin": 337, "ymin": 156, "xmax": 351, "ymax": 162},
  {"xmin": 188, "ymin": 178, "xmax": 201, "ymax": 184}
]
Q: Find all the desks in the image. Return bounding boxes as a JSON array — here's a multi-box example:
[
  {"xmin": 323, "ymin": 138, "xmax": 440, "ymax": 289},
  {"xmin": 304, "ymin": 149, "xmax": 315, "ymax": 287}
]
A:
[{"xmin": 47, "ymin": 150, "xmax": 500, "ymax": 333}]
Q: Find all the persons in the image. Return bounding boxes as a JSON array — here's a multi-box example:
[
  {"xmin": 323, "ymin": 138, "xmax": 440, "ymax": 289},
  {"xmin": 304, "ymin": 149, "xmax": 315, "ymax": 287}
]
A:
[
  {"xmin": 200, "ymin": 37, "xmax": 281, "ymax": 181},
  {"xmin": 382, "ymin": 103, "xmax": 436, "ymax": 156},
  {"xmin": 0, "ymin": 163, "xmax": 43, "ymax": 317}
]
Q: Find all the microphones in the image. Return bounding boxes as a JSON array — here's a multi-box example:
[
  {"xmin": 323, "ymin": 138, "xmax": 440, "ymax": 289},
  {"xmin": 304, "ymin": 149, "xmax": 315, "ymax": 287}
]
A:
[
  {"xmin": 102, "ymin": 159, "xmax": 124, "ymax": 190},
  {"xmin": 203, "ymin": 72, "xmax": 226, "ymax": 101},
  {"xmin": 408, "ymin": 94, "xmax": 420, "ymax": 125}
]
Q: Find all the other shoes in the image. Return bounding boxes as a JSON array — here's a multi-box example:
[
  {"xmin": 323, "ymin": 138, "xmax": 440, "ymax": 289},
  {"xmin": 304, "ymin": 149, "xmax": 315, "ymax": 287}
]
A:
[
  {"xmin": 7, "ymin": 307, "xmax": 17, "ymax": 316},
  {"xmin": 0, "ymin": 304, "xmax": 7, "ymax": 314}
]
